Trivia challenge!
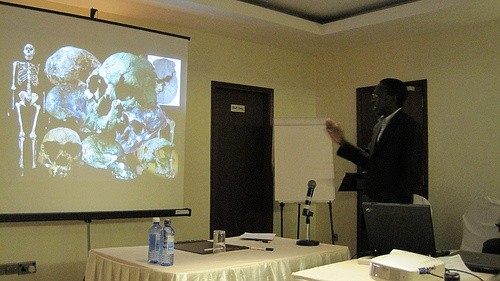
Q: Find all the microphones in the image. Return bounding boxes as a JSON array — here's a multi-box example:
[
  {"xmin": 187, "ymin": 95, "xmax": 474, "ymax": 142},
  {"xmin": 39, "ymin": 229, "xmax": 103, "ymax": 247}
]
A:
[{"xmin": 303, "ymin": 180, "xmax": 317, "ymax": 216}]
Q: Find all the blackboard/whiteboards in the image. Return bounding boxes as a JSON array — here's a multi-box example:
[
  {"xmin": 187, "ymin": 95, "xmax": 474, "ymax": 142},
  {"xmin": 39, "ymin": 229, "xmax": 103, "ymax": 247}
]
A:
[{"xmin": 274, "ymin": 118, "xmax": 335, "ymax": 203}]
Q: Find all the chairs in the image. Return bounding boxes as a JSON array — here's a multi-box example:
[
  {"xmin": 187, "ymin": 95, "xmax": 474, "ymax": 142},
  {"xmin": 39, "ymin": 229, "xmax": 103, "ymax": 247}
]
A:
[{"xmin": 460, "ymin": 203, "xmax": 500, "ymax": 255}]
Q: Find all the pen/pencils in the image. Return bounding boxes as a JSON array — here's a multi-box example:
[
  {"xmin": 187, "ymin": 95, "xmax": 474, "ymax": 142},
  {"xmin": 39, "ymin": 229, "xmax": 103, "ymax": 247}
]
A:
[
  {"xmin": 204, "ymin": 247, "xmax": 223, "ymax": 251},
  {"xmin": 252, "ymin": 247, "xmax": 274, "ymax": 252}
]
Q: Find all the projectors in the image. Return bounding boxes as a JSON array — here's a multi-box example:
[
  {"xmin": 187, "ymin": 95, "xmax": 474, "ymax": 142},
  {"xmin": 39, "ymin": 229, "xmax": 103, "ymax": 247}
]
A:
[{"xmin": 369, "ymin": 249, "xmax": 446, "ymax": 281}]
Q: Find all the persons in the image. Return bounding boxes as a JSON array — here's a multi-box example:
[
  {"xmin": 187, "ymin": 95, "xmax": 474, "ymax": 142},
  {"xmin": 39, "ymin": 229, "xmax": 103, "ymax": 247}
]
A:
[{"xmin": 326, "ymin": 78, "xmax": 425, "ymax": 256}]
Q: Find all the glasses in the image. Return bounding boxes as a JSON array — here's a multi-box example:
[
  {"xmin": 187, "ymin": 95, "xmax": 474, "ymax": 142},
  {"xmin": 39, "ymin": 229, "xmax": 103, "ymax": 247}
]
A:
[{"xmin": 371, "ymin": 93, "xmax": 384, "ymax": 98}]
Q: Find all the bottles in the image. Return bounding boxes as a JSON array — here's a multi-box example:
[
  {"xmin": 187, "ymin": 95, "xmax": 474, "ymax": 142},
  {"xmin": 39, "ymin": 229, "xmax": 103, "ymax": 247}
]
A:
[{"xmin": 147, "ymin": 217, "xmax": 175, "ymax": 266}]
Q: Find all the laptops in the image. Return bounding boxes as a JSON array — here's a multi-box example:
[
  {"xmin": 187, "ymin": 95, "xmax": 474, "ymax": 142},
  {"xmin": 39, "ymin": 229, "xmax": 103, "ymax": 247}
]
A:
[
  {"xmin": 450, "ymin": 250, "xmax": 500, "ymax": 274},
  {"xmin": 361, "ymin": 202, "xmax": 451, "ymax": 258}
]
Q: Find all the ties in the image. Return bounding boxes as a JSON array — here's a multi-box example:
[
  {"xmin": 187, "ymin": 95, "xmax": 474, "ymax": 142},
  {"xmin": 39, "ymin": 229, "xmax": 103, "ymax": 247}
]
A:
[{"xmin": 368, "ymin": 118, "xmax": 383, "ymax": 157}]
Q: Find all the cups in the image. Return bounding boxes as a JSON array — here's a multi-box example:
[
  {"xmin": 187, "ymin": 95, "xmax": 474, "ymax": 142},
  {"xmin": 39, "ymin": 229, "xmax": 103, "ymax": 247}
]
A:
[{"xmin": 213, "ymin": 229, "xmax": 227, "ymax": 254}]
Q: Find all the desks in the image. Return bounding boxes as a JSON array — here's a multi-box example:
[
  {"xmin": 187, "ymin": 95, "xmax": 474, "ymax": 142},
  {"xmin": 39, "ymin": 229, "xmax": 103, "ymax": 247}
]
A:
[
  {"xmin": 291, "ymin": 254, "xmax": 500, "ymax": 281},
  {"xmin": 85, "ymin": 234, "xmax": 350, "ymax": 281}
]
